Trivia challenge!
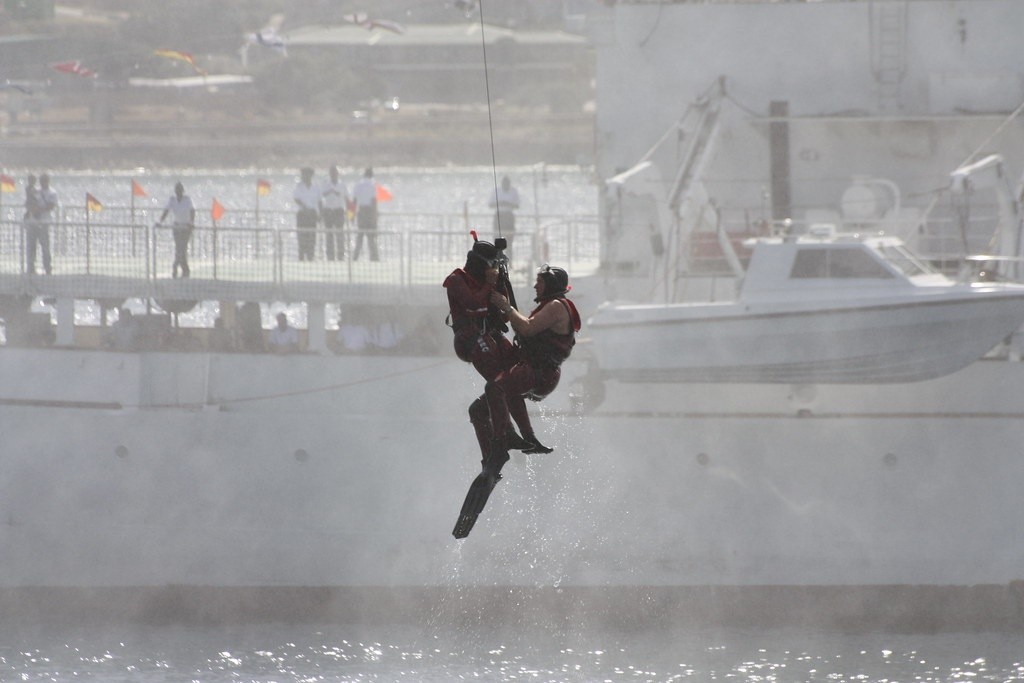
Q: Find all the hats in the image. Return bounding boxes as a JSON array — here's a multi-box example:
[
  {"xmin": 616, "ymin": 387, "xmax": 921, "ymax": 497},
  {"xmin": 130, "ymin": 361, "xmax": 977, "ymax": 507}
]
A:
[
  {"xmin": 473, "ymin": 240, "xmax": 499, "ymax": 275},
  {"xmin": 537, "ymin": 267, "xmax": 568, "ymax": 300}
]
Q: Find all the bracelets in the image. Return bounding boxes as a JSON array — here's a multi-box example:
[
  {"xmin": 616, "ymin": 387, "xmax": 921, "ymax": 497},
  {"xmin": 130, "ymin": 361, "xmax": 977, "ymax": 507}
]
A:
[{"xmin": 505, "ymin": 306, "xmax": 513, "ymax": 313}]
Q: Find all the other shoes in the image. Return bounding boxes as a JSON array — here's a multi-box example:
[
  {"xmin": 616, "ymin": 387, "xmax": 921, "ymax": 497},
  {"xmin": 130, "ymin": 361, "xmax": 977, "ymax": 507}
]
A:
[
  {"xmin": 507, "ymin": 433, "xmax": 535, "ymax": 451},
  {"xmin": 522, "ymin": 437, "xmax": 553, "ymax": 455},
  {"xmin": 481, "ymin": 442, "xmax": 510, "ymax": 482}
]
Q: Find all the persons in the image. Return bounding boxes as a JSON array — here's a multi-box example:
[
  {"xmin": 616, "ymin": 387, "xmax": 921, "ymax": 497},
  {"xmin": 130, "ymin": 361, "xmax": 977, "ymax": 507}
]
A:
[
  {"xmin": 443, "ymin": 240, "xmax": 552, "ymax": 454},
  {"xmin": 452, "ymin": 265, "xmax": 580, "ymax": 538},
  {"xmin": 352, "ymin": 167, "xmax": 380, "ymax": 262},
  {"xmin": 159, "ymin": 183, "xmax": 195, "ymax": 278},
  {"xmin": 294, "ymin": 168, "xmax": 322, "ymax": 261},
  {"xmin": 320, "ymin": 166, "xmax": 349, "ymax": 259},
  {"xmin": 27, "ymin": 173, "xmax": 58, "ymax": 273},
  {"xmin": 489, "ymin": 178, "xmax": 519, "ymax": 266}
]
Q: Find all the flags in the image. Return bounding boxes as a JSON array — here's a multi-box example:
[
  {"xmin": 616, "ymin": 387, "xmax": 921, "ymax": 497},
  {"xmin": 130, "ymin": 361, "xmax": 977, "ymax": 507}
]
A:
[
  {"xmin": 10, "ymin": 85, "xmax": 35, "ymax": 96},
  {"xmin": 52, "ymin": 61, "xmax": 97, "ymax": 77},
  {"xmin": 0, "ymin": 175, "xmax": 16, "ymax": 193},
  {"xmin": 133, "ymin": 182, "xmax": 145, "ymax": 195},
  {"xmin": 213, "ymin": 201, "xmax": 224, "ymax": 220},
  {"xmin": 246, "ymin": 33, "xmax": 285, "ymax": 56},
  {"xmin": 344, "ymin": 13, "xmax": 403, "ymax": 33},
  {"xmin": 378, "ymin": 183, "xmax": 390, "ymax": 200},
  {"xmin": 157, "ymin": 50, "xmax": 202, "ymax": 72},
  {"xmin": 259, "ymin": 179, "xmax": 270, "ymax": 195},
  {"xmin": 88, "ymin": 194, "xmax": 102, "ymax": 211}
]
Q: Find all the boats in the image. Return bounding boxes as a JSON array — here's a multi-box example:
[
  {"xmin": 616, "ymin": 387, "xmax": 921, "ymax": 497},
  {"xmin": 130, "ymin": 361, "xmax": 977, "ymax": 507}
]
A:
[
  {"xmin": 0, "ymin": 1, "xmax": 1024, "ymax": 639},
  {"xmin": 587, "ymin": 226, "xmax": 1023, "ymax": 388}
]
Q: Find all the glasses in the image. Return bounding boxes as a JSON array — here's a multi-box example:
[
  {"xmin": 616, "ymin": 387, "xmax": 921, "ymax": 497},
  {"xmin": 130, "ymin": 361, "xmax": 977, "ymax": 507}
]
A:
[
  {"xmin": 537, "ymin": 263, "xmax": 551, "ymax": 273},
  {"xmin": 488, "ymin": 259, "xmax": 506, "ymax": 269}
]
